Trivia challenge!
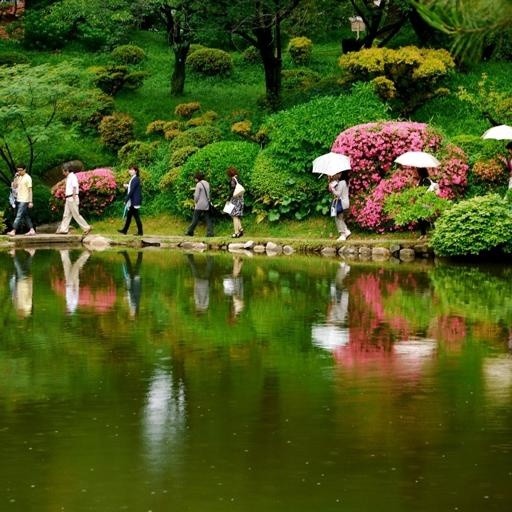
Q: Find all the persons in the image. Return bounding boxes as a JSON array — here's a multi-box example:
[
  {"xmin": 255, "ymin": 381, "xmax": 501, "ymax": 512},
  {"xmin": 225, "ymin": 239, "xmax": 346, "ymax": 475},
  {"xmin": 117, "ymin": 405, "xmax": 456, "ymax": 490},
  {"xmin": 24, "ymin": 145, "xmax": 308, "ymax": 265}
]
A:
[
  {"xmin": 7, "ymin": 248, "xmax": 143, "ymax": 329},
  {"xmin": 416, "ymin": 168, "xmax": 431, "ymax": 185},
  {"xmin": 185, "ymin": 172, "xmax": 214, "ymax": 237},
  {"xmin": 223, "ymin": 167, "xmax": 246, "ymax": 238},
  {"xmin": 4, "ymin": 161, "xmax": 142, "ymax": 236},
  {"xmin": 326, "ymin": 261, "xmax": 351, "ymax": 328},
  {"xmin": 223, "ymin": 253, "xmax": 244, "ymax": 326},
  {"xmin": 497, "ymin": 141, "xmax": 512, "ymax": 189},
  {"xmin": 183, "ymin": 254, "xmax": 212, "ymax": 316},
  {"xmin": 329, "ymin": 169, "xmax": 352, "ymax": 240}
]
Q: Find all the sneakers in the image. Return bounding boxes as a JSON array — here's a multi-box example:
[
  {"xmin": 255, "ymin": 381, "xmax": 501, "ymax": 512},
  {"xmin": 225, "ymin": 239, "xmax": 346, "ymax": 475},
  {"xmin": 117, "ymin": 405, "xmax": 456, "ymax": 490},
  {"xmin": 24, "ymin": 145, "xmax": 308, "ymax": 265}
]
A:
[
  {"xmin": 337, "ymin": 230, "xmax": 351, "ymax": 241},
  {"xmin": 183, "ymin": 232, "xmax": 214, "ymax": 236},
  {"xmin": 8, "ymin": 230, "xmax": 35, "ymax": 236}
]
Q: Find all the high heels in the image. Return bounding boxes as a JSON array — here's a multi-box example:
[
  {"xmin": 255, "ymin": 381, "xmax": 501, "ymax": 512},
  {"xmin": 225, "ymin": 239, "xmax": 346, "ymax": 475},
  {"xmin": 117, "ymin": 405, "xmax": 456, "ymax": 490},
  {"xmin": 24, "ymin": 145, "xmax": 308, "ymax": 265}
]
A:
[{"xmin": 232, "ymin": 228, "xmax": 243, "ymax": 238}]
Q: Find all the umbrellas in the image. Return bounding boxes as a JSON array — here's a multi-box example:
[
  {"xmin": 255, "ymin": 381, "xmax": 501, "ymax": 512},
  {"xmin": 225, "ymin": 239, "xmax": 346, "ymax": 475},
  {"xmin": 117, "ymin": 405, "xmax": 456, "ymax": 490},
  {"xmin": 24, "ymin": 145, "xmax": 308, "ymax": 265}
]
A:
[
  {"xmin": 394, "ymin": 152, "xmax": 441, "ymax": 167},
  {"xmin": 311, "ymin": 323, "xmax": 350, "ymax": 352},
  {"xmin": 312, "ymin": 152, "xmax": 352, "ymax": 176},
  {"xmin": 480, "ymin": 125, "xmax": 512, "ymax": 154}
]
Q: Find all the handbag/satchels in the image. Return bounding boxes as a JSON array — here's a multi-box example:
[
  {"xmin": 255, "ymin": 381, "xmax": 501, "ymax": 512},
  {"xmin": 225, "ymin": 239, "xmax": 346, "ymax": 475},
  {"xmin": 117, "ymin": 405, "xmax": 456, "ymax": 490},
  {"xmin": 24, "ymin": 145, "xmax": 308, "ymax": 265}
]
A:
[
  {"xmin": 209, "ymin": 202, "xmax": 216, "ymax": 217},
  {"xmin": 223, "ymin": 184, "xmax": 245, "ymax": 214},
  {"xmin": 427, "ymin": 182, "xmax": 441, "ymax": 195},
  {"xmin": 331, "ymin": 198, "xmax": 343, "ymax": 217}
]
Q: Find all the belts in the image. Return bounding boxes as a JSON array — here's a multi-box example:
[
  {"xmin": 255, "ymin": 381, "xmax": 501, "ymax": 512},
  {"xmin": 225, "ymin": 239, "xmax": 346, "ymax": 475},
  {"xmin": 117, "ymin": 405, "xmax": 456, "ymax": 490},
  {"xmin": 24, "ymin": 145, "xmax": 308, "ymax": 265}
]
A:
[{"xmin": 65, "ymin": 194, "xmax": 78, "ymax": 198}]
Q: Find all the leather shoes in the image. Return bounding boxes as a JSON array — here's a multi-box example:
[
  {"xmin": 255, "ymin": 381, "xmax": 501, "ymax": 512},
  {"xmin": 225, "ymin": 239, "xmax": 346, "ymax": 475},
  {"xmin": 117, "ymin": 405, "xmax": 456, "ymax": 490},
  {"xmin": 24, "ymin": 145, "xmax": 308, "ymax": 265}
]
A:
[{"xmin": 117, "ymin": 230, "xmax": 143, "ymax": 235}]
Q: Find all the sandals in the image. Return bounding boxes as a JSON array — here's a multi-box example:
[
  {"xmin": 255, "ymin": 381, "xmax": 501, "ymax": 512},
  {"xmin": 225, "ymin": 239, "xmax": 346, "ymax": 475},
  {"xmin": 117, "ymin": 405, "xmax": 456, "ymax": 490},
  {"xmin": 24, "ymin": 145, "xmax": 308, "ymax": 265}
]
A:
[{"xmin": 56, "ymin": 225, "xmax": 92, "ymax": 235}]
